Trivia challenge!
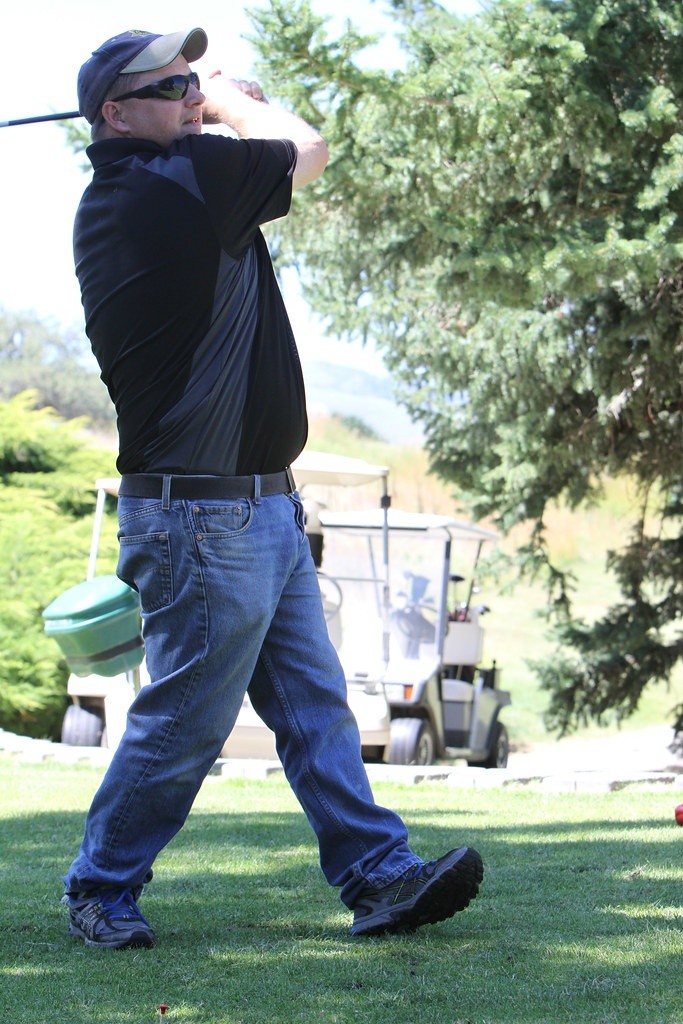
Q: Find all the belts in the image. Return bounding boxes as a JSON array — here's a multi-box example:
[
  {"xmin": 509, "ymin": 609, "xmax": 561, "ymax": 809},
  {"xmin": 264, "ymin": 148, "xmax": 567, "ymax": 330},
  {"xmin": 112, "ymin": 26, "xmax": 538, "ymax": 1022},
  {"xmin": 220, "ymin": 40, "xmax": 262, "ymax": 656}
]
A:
[{"xmin": 118, "ymin": 466, "xmax": 296, "ymax": 498}]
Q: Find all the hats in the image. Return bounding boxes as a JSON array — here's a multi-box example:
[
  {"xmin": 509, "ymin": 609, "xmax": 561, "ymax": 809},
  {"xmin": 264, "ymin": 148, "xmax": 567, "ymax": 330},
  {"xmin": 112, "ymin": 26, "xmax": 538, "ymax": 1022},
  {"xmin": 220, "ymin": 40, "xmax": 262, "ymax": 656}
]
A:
[{"xmin": 76, "ymin": 27, "xmax": 214, "ymax": 125}]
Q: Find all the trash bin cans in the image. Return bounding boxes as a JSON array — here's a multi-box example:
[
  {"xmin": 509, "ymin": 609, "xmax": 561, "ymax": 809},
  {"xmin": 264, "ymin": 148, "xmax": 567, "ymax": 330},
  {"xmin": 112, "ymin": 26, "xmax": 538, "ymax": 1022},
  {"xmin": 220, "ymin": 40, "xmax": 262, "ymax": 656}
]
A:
[{"xmin": 40, "ymin": 574, "xmax": 145, "ymax": 677}]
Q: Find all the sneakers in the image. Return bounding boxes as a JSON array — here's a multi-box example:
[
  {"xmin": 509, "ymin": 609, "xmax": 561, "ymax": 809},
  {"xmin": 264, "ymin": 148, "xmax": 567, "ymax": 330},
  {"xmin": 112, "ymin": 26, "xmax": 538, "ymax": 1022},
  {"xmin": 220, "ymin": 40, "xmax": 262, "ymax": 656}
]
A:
[
  {"xmin": 69, "ymin": 888, "xmax": 156, "ymax": 949},
  {"xmin": 349, "ymin": 848, "xmax": 483, "ymax": 936}
]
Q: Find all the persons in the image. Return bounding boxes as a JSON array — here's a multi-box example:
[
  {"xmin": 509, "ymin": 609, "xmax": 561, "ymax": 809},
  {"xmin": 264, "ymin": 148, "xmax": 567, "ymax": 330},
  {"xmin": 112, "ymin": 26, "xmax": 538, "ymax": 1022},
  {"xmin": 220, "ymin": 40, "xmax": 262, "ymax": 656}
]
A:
[{"xmin": 62, "ymin": 28, "xmax": 485, "ymax": 950}]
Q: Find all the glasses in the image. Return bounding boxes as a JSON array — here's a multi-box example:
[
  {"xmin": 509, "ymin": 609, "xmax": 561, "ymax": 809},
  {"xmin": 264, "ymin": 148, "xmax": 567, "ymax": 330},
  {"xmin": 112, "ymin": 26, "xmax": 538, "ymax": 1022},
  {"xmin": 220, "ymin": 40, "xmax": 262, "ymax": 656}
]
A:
[{"xmin": 111, "ymin": 72, "xmax": 208, "ymax": 104}]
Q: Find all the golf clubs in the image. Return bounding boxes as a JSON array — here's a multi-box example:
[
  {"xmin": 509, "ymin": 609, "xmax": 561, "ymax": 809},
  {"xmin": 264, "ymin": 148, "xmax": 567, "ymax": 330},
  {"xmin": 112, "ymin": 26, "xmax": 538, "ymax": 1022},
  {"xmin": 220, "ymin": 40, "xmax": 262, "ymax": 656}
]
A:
[{"xmin": 0, "ymin": 111, "xmax": 86, "ymax": 128}]
